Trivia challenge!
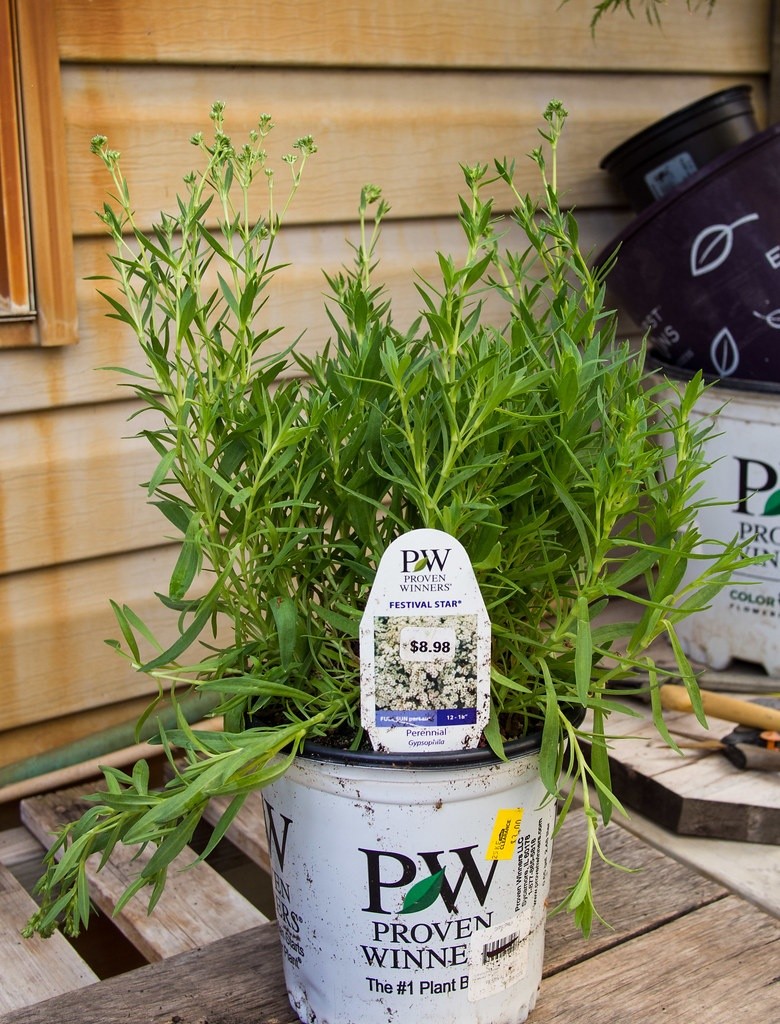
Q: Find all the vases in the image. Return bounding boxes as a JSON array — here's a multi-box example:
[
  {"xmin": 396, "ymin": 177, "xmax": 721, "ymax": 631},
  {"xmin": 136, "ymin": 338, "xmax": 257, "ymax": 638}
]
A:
[{"xmin": 586, "ymin": 83, "xmax": 779, "ymax": 682}]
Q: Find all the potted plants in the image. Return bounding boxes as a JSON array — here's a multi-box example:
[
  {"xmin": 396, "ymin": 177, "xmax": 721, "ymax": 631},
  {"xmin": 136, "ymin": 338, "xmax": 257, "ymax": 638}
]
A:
[{"xmin": 22, "ymin": 95, "xmax": 779, "ymax": 1024}]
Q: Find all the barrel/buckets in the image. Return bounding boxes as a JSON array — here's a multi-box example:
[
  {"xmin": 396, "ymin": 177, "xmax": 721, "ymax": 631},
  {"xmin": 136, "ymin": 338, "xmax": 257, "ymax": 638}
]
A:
[
  {"xmin": 591, "ymin": 125, "xmax": 779, "ymax": 384},
  {"xmin": 598, "ymin": 86, "xmax": 758, "ymax": 214}
]
